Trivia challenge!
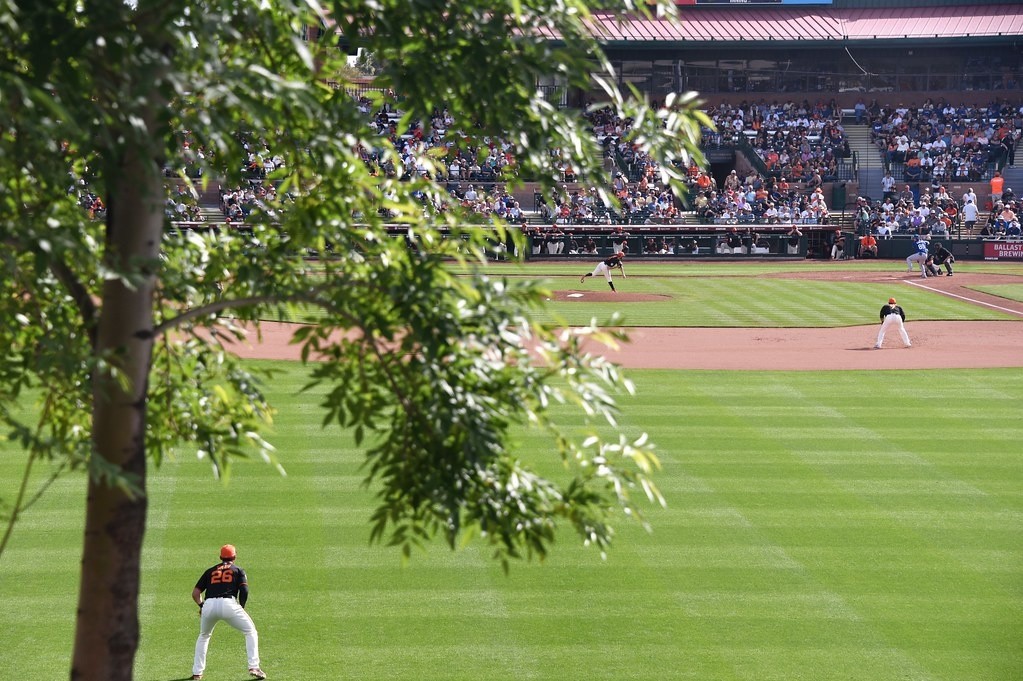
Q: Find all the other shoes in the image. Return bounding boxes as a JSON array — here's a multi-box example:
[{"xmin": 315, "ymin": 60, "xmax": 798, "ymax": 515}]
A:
[
  {"xmin": 874, "ymin": 345, "xmax": 880, "ymax": 348},
  {"xmin": 904, "ymin": 344, "xmax": 911, "ymax": 348},
  {"xmin": 947, "ymin": 274, "xmax": 953, "ymax": 276},
  {"xmin": 938, "ymin": 272, "xmax": 943, "ymax": 274},
  {"xmin": 613, "ymin": 289, "xmax": 616, "ymax": 292},
  {"xmin": 581, "ymin": 275, "xmax": 585, "ymax": 283}
]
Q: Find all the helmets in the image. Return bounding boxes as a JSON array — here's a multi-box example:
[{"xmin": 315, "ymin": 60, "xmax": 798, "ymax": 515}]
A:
[{"xmin": 911, "ymin": 234, "xmax": 920, "ymax": 242}]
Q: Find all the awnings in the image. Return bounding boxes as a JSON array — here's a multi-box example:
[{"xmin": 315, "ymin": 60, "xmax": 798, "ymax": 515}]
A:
[{"xmin": 304, "ymin": 3, "xmax": 1023, "ymax": 48}]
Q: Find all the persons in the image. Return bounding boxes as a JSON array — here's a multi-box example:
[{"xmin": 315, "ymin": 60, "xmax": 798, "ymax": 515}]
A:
[
  {"xmin": 930, "ymin": 242, "xmax": 954, "ymax": 277},
  {"xmin": 60, "ymin": 87, "xmax": 1023, "ymax": 261},
  {"xmin": 906, "ymin": 234, "xmax": 930, "ymax": 278},
  {"xmin": 581, "ymin": 252, "xmax": 626, "ymax": 294},
  {"xmin": 917, "ymin": 253, "xmax": 944, "ymax": 276},
  {"xmin": 874, "ymin": 298, "xmax": 911, "ymax": 348},
  {"xmin": 192, "ymin": 544, "xmax": 266, "ymax": 679}
]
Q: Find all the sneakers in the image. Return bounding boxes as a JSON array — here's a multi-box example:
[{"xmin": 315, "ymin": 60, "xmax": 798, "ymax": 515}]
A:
[
  {"xmin": 192, "ymin": 675, "xmax": 203, "ymax": 679},
  {"xmin": 249, "ymin": 668, "xmax": 266, "ymax": 679}
]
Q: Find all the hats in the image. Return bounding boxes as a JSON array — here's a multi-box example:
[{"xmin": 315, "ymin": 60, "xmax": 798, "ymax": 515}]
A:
[
  {"xmin": 220, "ymin": 544, "xmax": 235, "ymax": 558},
  {"xmin": 623, "ymin": 241, "xmax": 628, "ymax": 245},
  {"xmin": 553, "ymin": 224, "xmax": 558, "ymax": 228},
  {"xmin": 535, "ymin": 227, "xmax": 540, "ymax": 231},
  {"xmin": 618, "ymin": 252, "xmax": 625, "ymax": 257},
  {"xmin": 617, "ymin": 227, "xmax": 622, "ymax": 231},
  {"xmin": 888, "ymin": 298, "xmax": 896, "ymax": 304}
]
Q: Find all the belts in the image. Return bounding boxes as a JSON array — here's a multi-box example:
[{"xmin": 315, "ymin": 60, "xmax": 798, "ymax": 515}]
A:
[{"xmin": 216, "ymin": 595, "xmax": 232, "ymax": 598}]
